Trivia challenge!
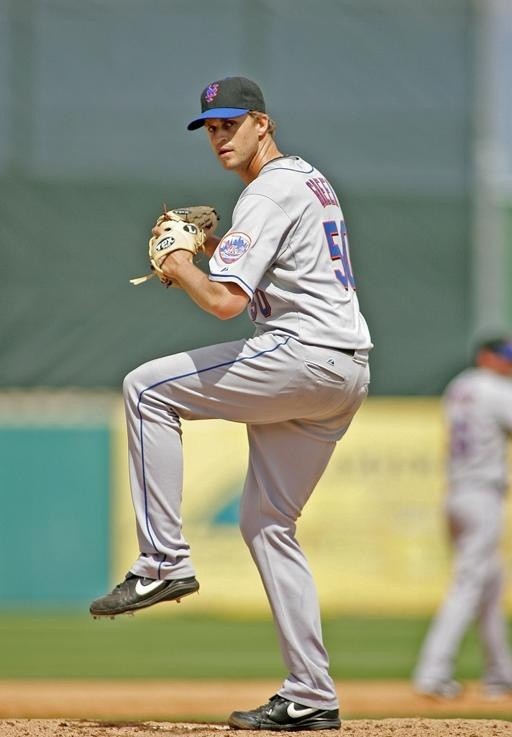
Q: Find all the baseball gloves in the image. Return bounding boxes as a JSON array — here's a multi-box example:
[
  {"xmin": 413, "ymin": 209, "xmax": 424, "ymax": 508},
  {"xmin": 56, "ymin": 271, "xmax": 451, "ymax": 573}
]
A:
[{"xmin": 149, "ymin": 205, "xmax": 220, "ymax": 288}]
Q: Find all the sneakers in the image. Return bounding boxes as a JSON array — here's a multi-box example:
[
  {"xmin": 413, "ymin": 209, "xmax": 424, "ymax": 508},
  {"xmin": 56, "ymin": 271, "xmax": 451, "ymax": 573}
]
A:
[
  {"xmin": 88, "ymin": 567, "xmax": 201, "ymax": 616},
  {"xmin": 225, "ymin": 688, "xmax": 342, "ymax": 731}
]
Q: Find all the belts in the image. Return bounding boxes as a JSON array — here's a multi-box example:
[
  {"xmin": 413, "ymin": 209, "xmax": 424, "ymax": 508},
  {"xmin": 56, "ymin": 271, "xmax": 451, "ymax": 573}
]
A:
[{"xmin": 308, "ymin": 335, "xmax": 358, "ymax": 359}]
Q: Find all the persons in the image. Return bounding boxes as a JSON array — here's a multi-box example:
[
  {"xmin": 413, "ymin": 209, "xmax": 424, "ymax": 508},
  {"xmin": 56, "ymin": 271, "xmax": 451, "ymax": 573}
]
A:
[
  {"xmin": 89, "ymin": 77, "xmax": 374, "ymax": 728},
  {"xmin": 412, "ymin": 335, "xmax": 512, "ymax": 702}
]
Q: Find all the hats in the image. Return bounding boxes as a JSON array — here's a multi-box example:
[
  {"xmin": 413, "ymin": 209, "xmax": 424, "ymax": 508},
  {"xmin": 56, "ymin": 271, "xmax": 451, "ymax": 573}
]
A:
[{"xmin": 185, "ymin": 72, "xmax": 268, "ymax": 131}]
